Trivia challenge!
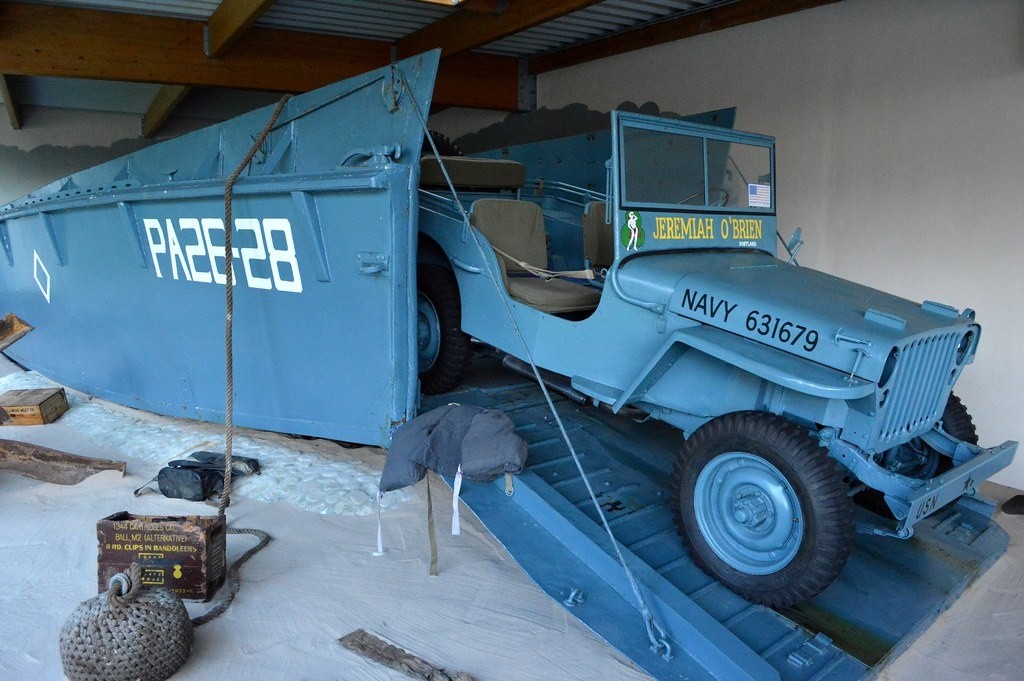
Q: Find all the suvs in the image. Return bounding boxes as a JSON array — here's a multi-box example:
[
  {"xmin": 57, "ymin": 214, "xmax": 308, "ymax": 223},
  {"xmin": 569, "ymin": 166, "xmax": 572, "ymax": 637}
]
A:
[{"xmin": 414, "ymin": 110, "xmax": 1018, "ymax": 607}]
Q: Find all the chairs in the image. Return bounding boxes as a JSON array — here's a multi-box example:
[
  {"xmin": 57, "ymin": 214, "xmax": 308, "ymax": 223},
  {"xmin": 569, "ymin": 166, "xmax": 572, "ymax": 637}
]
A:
[
  {"xmin": 582, "ymin": 200, "xmax": 614, "ymax": 287},
  {"xmin": 470, "ymin": 197, "xmax": 601, "ymax": 315}
]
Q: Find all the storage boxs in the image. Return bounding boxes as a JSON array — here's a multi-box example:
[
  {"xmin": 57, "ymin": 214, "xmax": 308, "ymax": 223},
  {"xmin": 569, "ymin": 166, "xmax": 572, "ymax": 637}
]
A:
[
  {"xmin": 0, "ymin": 387, "xmax": 70, "ymax": 426},
  {"xmin": 96, "ymin": 510, "xmax": 226, "ymax": 604}
]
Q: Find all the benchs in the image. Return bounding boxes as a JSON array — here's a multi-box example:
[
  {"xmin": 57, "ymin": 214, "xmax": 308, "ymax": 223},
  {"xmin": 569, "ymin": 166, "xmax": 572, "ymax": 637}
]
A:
[{"xmin": 420, "ymin": 155, "xmax": 551, "ymax": 250}]
